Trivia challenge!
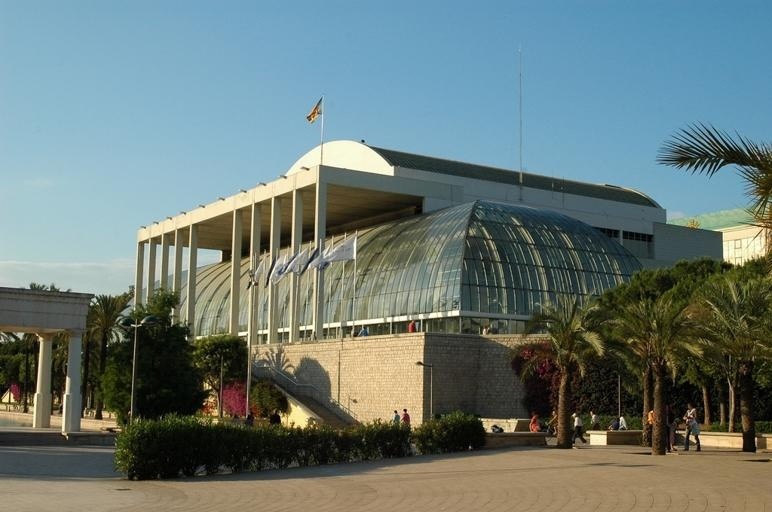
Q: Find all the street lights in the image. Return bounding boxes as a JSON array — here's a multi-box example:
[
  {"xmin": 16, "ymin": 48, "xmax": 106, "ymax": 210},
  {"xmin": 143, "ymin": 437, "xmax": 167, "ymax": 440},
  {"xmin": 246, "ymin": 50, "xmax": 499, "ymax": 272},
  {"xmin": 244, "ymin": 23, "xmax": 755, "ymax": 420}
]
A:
[
  {"xmin": 493, "ymin": 299, "xmax": 508, "ymax": 334},
  {"xmin": 533, "ymin": 302, "xmax": 549, "ymax": 314},
  {"xmin": 21, "ymin": 332, "xmax": 35, "ymax": 415},
  {"xmin": 130, "ymin": 315, "xmax": 156, "ymax": 418},
  {"xmin": 414, "ymin": 358, "xmax": 435, "ymax": 421}
]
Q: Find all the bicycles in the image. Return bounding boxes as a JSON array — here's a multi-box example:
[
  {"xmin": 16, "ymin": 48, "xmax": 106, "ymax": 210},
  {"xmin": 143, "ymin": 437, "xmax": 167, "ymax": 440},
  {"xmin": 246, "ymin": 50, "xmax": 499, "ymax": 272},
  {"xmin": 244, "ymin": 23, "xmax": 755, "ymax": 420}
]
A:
[{"xmin": 538, "ymin": 423, "xmax": 559, "ymax": 443}]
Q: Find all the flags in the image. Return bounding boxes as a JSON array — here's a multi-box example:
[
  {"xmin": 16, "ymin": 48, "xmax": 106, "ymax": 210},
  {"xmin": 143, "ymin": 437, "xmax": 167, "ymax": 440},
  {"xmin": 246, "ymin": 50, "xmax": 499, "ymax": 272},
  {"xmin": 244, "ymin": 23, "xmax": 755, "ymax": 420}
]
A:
[
  {"xmin": 306, "ymin": 96, "xmax": 323, "ymax": 123},
  {"xmin": 247, "ymin": 239, "xmax": 356, "ymax": 291}
]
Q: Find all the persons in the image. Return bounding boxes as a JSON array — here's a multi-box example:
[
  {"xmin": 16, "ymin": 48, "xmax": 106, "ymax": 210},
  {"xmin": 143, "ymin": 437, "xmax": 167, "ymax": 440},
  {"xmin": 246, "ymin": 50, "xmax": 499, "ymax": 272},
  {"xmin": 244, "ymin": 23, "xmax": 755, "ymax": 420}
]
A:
[
  {"xmin": 358, "ymin": 324, "xmax": 370, "ymax": 336},
  {"xmin": 268, "ymin": 408, "xmax": 280, "ymax": 425},
  {"xmin": 487, "ymin": 323, "xmax": 498, "ymax": 335},
  {"xmin": 391, "ymin": 410, "xmax": 399, "ymax": 425},
  {"xmin": 309, "ymin": 331, "xmax": 317, "ymax": 341},
  {"xmin": 245, "ymin": 411, "xmax": 253, "ymax": 425},
  {"xmin": 409, "ymin": 320, "xmax": 416, "ymax": 333},
  {"xmin": 399, "ymin": 408, "xmax": 411, "ymax": 428},
  {"xmin": 529, "ymin": 401, "xmax": 702, "ymax": 454},
  {"xmin": 482, "ymin": 324, "xmax": 489, "ymax": 335}
]
